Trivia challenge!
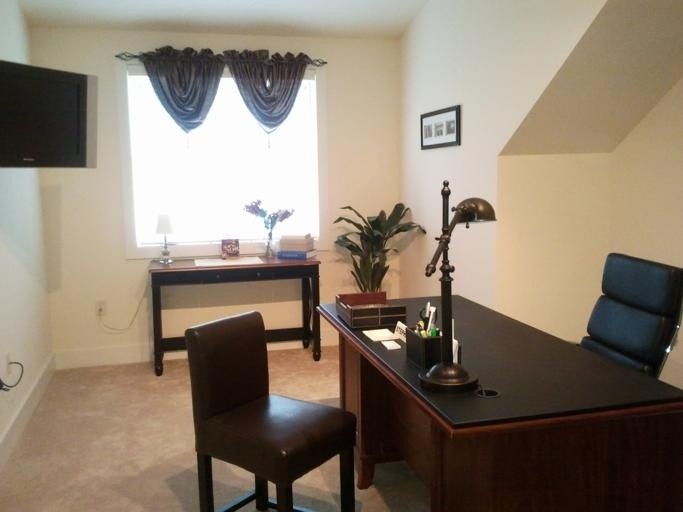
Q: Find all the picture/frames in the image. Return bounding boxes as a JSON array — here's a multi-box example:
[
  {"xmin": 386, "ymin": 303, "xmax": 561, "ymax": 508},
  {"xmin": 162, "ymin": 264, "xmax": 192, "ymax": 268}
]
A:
[{"xmin": 419, "ymin": 104, "xmax": 462, "ymax": 152}]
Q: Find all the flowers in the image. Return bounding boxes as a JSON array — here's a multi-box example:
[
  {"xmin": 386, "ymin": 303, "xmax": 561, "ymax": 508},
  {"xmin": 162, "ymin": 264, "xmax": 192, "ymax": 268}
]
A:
[{"xmin": 242, "ymin": 196, "xmax": 296, "ymax": 230}]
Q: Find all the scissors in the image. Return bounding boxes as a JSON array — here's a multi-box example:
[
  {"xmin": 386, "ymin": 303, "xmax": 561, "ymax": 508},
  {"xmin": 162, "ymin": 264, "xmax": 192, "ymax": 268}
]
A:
[{"xmin": 419, "ymin": 307, "xmax": 437, "ymax": 331}]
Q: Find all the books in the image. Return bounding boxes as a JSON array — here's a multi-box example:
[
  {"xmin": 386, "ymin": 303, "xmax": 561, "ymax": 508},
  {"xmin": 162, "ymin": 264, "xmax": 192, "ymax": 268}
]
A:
[{"xmin": 277, "ymin": 249, "xmax": 317, "ymax": 260}]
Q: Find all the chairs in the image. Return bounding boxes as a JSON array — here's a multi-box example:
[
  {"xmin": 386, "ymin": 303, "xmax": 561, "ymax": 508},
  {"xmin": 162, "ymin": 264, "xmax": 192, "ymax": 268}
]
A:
[
  {"xmin": 573, "ymin": 247, "xmax": 682, "ymax": 380},
  {"xmin": 185, "ymin": 305, "xmax": 362, "ymax": 512}
]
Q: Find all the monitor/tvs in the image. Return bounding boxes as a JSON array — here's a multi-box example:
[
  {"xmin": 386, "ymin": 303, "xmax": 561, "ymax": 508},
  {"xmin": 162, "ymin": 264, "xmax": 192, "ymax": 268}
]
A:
[{"xmin": 0, "ymin": 61, "xmax": 87, "ymax": 167}]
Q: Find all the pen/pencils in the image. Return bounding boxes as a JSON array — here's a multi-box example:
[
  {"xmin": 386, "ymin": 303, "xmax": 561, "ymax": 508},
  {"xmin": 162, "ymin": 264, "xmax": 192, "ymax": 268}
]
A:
[{"xmin": 414, "ymin": 301, "xmax": 443, "ymax": 338}]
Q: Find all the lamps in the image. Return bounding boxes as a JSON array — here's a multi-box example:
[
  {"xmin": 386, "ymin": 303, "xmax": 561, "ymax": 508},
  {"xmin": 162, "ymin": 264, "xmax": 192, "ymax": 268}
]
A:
[{"xmin": 417, "ymin": 177, "xmax": 499, "ymax": 395}]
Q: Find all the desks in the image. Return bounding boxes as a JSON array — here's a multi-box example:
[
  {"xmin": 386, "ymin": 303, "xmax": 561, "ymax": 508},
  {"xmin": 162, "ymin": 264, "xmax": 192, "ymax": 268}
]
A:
[
  {"xmin": 315, "ymin": 292, "xmax": 682, "ymax": 509},
  {"xmin": 146, "ymin": 253, "xmax": 323, "ymax": 378}
]
